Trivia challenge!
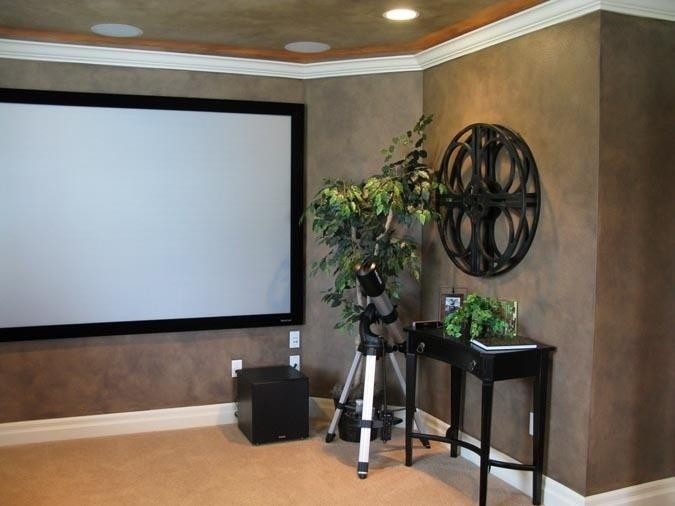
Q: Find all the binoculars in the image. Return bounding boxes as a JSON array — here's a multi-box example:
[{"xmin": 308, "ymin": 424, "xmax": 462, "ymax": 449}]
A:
[{"xmin": 325, "ymin": 255, "xmax": 432, "ymax": 479}]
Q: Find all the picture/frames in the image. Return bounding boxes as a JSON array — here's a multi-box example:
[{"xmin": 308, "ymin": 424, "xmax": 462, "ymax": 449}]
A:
[{"xmin": 440, "ymin": 294, "xmax": 464, "ymax": 322}]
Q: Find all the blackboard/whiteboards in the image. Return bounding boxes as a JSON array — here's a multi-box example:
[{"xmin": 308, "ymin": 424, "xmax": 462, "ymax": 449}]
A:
[{"xmin": 0, "ymin": 88, "xmax": 308, "ymax": 343}]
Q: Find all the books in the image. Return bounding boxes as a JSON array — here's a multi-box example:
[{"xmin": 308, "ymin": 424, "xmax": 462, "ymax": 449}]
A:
[{"xmin": 472, "ymin": 335, "xmax": 538, "ymax": 350}]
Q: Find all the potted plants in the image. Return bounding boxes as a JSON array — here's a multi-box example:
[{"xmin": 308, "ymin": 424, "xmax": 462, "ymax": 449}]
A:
[{"xmin": 299, "ymin": 112, "xmax": 454, "ymax": 442}]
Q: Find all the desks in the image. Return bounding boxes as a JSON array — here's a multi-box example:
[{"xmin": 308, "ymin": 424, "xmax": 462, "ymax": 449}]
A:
[{"xmin": 403, "ymin": 320, "xmax": 557, "ymax": 506}]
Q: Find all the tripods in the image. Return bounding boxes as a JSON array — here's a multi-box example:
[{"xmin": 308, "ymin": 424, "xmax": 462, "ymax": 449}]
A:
[{"xmin": 326, "ymin": 336, "xmax": 433, "ymax": 481}]
